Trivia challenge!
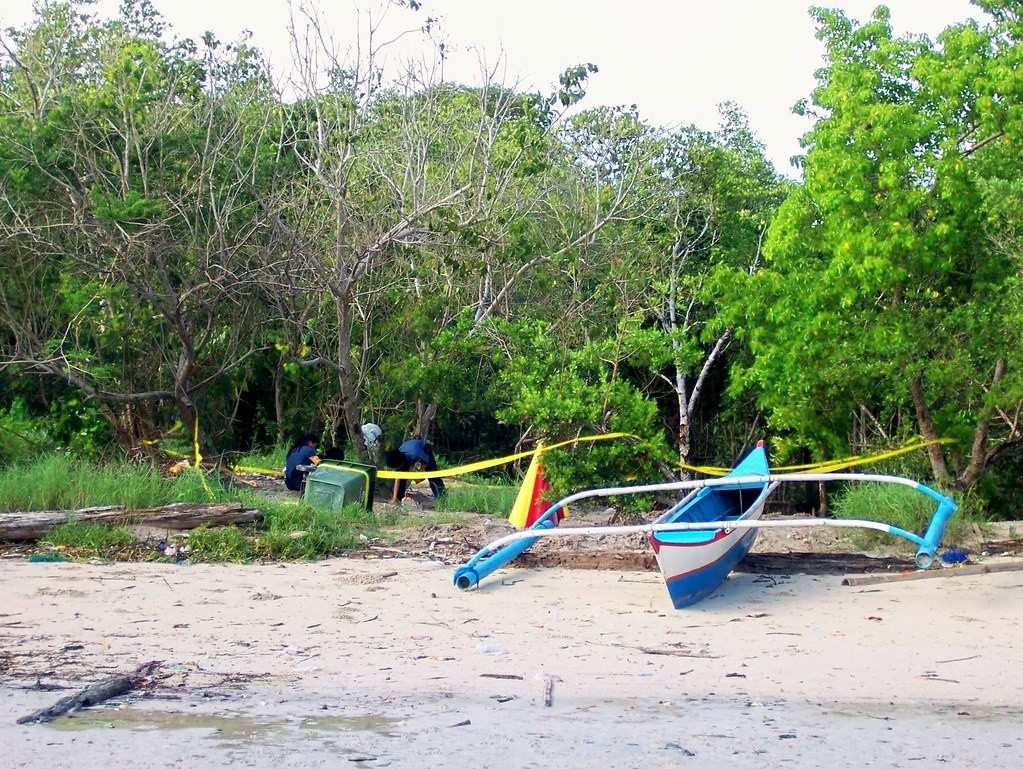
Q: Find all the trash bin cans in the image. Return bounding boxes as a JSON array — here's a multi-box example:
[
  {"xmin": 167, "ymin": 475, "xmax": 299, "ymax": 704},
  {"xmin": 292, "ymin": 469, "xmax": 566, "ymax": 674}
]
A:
[{"xmin": 295, "ymin": 459, "xmax": 377, "ymax": 513}]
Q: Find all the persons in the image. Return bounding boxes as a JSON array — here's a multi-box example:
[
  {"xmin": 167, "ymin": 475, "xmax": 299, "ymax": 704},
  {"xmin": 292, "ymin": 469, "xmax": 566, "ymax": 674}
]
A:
[
  {"xmin": 361, "ymin": 422, "xmax": 382, "ymax": 464},
  {"xmin": 386, "ymin": 438, "xmax": 447, "ymax": 504},
  {"xmin": 285, "ymin": 433, "xmax": 344, "ymax": 492}
]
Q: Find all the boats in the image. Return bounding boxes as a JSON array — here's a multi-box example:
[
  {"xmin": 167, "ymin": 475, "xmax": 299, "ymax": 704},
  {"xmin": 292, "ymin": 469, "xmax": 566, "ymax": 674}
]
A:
[{"xmin": 649, "ymin": 441, "xmax": 778, "ymax": 609}]
